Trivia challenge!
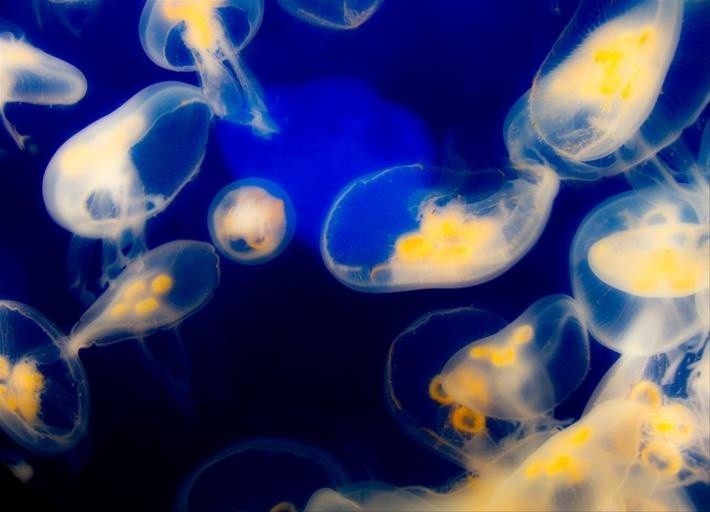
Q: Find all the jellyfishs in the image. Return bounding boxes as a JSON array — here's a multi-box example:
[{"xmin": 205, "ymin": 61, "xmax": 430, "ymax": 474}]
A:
[{"xmin": 0, "ymin": 0, "xmax": 710, "ymax": 512}]
